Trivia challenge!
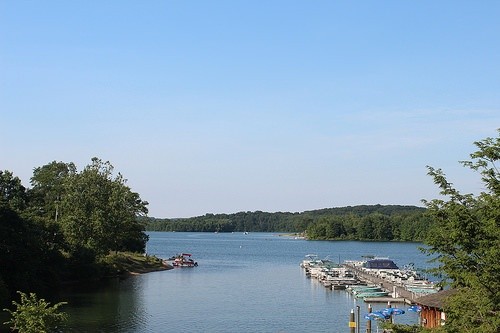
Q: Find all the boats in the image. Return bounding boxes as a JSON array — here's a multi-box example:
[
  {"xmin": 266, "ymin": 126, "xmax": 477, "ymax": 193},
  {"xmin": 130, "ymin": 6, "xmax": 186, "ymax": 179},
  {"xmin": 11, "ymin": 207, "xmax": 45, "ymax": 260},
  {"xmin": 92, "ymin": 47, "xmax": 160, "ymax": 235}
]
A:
[
  {"xmin": 347, "ymin": 258, "xmax": 443, "ymax": 293},
  {"xmin": 300, "ymin": 260, "xmax": 390, "ymax": 298},
  {"xmin": 172, "ymin": 253, "xmax": 194, "ymax": 267}
]
